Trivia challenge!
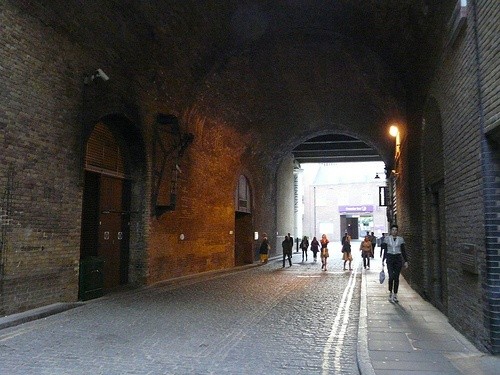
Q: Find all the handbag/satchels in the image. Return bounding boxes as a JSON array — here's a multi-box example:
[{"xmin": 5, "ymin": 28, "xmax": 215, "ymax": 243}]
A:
[{"xmin": 380, "ymin": 270, "xmax": 385, "ymax": 284}]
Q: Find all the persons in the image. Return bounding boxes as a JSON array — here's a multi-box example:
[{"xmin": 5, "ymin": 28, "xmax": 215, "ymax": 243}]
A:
[
  {"xmin": 281, "ymin": 236, "xmax": 292, "ymax": 268},
  {"xmin": 320, "ymin": 234, "xmax": 329, "ymax": 271},
  {"xmin": 366, "ymin": 232, "xmax": 376, "ymax": 258},
  {"xmin": 380, "ymin": 233, "xmax": 385, "ymax": 257},
  {"xmin": 287, "ymin": 233, "xmax": 293, "ymax": 259},
  {"xmin": 382, "ymin": 225, "xmax": 408, "ymax": 303},
  {"xmin": 341, "ymin": 232, "xmax": 354, "ymax": 270},
  {"xmin": 300, "ymin": 236, "xmax": 309, "ymax": 262},
  {"xmin": 311, "ymin": 237, "xmax": 320, "ymax": 261},
  {"xmin": 259, "ymin": 233, "xmax": 271, "ymax": 264},
  {"xmin": 360, "ymin": 236, "xmax": 373, "ymax": 269}
]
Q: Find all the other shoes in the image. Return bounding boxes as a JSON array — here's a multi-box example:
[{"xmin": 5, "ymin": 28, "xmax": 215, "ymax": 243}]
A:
[
  {"xmin": 305, "ymin": 259, "xmax": 308, "ymax": 261},
  {"xmin": 343, "ymin": 268, "xmax": 348, "ymax": 270},
  {"xmin": 363, "ymin": 267, "xmax": 366, "ymax": 269},
  {"xmin": 393, "ymin": 295, "xmax": 398, "ymax": 302},
  {"xmin": 388, "ymin": 294, "xmax": 393, "ymax": 301},
  {"xmin": 289, "ymin": 265, "xmax": 292, "ymax": 267},
  {"xmin": 349, "ymin": 268, "xmax": 353, "ymax": 271},
  {"xmin": 282, "ymin": 266, "xmax": 285, "ymax": 268},
  {"xmin": 322, "ymin": 263, "xmax": 325, "ymax": 269},
  {"xmin": 367, "ymin": 264, "xmax": 370, "ymax": 267},
  {"xmin": 314, "ymin": 258, "xmax": 317, "ymax": 261},
  {"xmin": 302, "ymin": 259, "xmax": 305, "ymax": 262}
]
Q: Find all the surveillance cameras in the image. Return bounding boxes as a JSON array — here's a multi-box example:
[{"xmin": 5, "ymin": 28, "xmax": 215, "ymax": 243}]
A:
[{"xmin": 92, "ymin": 69, "xmax": 110, "ymax": 83}]
